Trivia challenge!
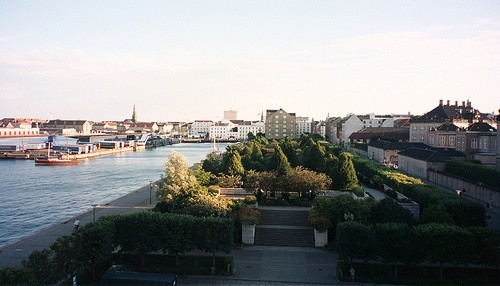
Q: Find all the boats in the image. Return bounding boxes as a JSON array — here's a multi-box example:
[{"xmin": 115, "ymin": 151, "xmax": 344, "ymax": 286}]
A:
[{"xmin": 34, "ymin": 144, "xmax": 80, "ymax": 164}]
